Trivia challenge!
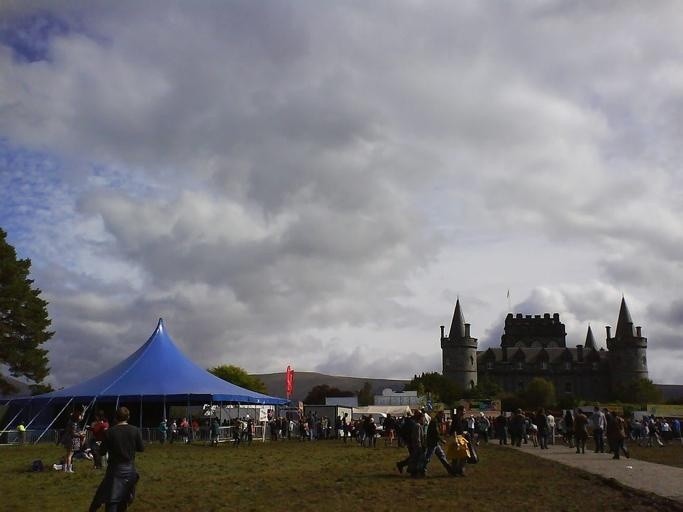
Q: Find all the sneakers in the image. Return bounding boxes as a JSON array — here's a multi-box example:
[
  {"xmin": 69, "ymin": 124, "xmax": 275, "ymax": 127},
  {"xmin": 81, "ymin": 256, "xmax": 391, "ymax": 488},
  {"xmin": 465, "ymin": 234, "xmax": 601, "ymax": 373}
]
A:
[{"xmin": 396, "ymin": 462, "xmax": 403, "ymax": 473}]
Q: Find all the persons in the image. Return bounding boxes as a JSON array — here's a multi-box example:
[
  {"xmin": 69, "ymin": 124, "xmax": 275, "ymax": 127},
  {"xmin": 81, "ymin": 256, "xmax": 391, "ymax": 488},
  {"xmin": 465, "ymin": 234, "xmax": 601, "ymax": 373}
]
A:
[
  {"xmin": 267, "ymin": 406, "xmax": 682, "ymax": 478},
  {"xmin": 209, "ymin": 416, "xmax": 256, "ymax": 448},
  {"xmin": 87, "ymin": 406, "xmax": 145, "ymax": 512},
  {"xmin": 157, "ymin": 416, "xmax": 200, "ymax": 446},
  {"xmin": 16, "ymin": 420, "xmax": 27, "ymax": 446},
  {"xmin": 86, "ymin": 408, "xmax": 110, "ymax": 468},
  {"xmin": 62, "ymin": 397, "xmax": 87, "ymax": 473}
]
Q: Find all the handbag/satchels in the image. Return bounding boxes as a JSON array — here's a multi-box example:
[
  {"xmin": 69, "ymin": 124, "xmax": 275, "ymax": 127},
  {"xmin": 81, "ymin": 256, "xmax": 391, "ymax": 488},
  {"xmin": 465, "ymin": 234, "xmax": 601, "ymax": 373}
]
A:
[
  {"xmin": 410, "ymin": 446, "xmax": 428, "ymax": 459},
  {"xmin": 116, "ymin": 465, "xmax": 139, "ymax": 486},
  {"xmin": 466, "ymin": 445, "xmax": 479, "ymax": 464},
  {"xmin": 541, "ymin": 427, "xmax": 549, "ymax": 436}
]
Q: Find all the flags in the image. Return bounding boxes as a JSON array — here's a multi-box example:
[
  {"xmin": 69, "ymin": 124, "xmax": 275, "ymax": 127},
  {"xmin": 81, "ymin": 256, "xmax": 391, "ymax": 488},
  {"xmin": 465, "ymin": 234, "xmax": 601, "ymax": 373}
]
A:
[{"xmin": 427, "ymin": 395, "xmax": 433, "ymax": 409}]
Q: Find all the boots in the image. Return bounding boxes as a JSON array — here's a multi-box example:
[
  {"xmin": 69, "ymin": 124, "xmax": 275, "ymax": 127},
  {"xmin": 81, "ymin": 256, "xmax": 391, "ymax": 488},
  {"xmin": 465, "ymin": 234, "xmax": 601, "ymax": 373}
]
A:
[{"xmin": 62, "ymin": 464, "xmax": 74, "ymax": 473}]
